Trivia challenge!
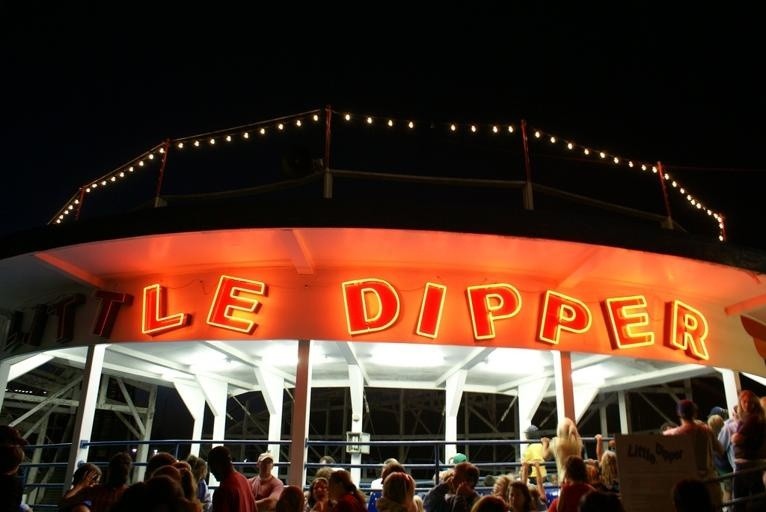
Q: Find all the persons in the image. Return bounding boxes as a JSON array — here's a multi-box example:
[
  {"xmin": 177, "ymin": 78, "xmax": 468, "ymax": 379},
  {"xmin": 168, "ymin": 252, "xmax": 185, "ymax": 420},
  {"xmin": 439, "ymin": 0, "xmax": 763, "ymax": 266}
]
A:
[
  {"xmin": 368, "ymin": 458, "xmax": 422, "ymax": 509},
  {"xmin": 542, "ymin": 417, "xmax": 583, "ymax": 485},
  {"xmin": 247, "ymin": 452, "xmax": 284, "ymax": 509},
  {"xmin": 2, "ymin": 425, "xmax": 24, "ymax": 509},
  {"xmin": 57, "ymin": 452, "xmax": 211, "ymax": 509},
  {"xmin": 595, "ymin": 434, "xmax": 617, "ymax": 461},
  {"xmin": 277, "ymin": 486, "xmax": 304, "ymax": 509},
  {"xmin": 207, "ymin": 446, "xmax": 256, "ymax": 509},
  {"xmin": 422, "ymin": 452, "xmax": 623, "ymax": 509},
  {"xmin": 663, "ymin": 390, "xmax": 763, "ymax": 509},
  {"xmin": 307, "ymin": 456, "xmax": 366, "ymax": 509},
  {"xmin": 520, "ymin": 426, "xmax": 548, "ymax": 484}
]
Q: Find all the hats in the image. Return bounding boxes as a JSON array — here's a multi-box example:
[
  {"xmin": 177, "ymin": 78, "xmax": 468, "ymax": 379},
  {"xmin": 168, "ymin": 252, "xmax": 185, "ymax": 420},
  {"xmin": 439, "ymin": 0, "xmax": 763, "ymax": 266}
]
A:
[
  {"xmin": 258, "ymin": 453, "xmax": 274, "ymax": 464},
  {"xmin": 0, "ymin": 425, "xmax": 27, "ymax": 445},
  {"xmin": 712, "ymin": 407, "xmax": 728, "ymax": 417},
  {"xmin": 453, "ymin": 454, "xmax": 467, "ymax": 464},
  {"xmin": 525, "ymin": 425, "xmax": 538, "ymax": 433}
]
